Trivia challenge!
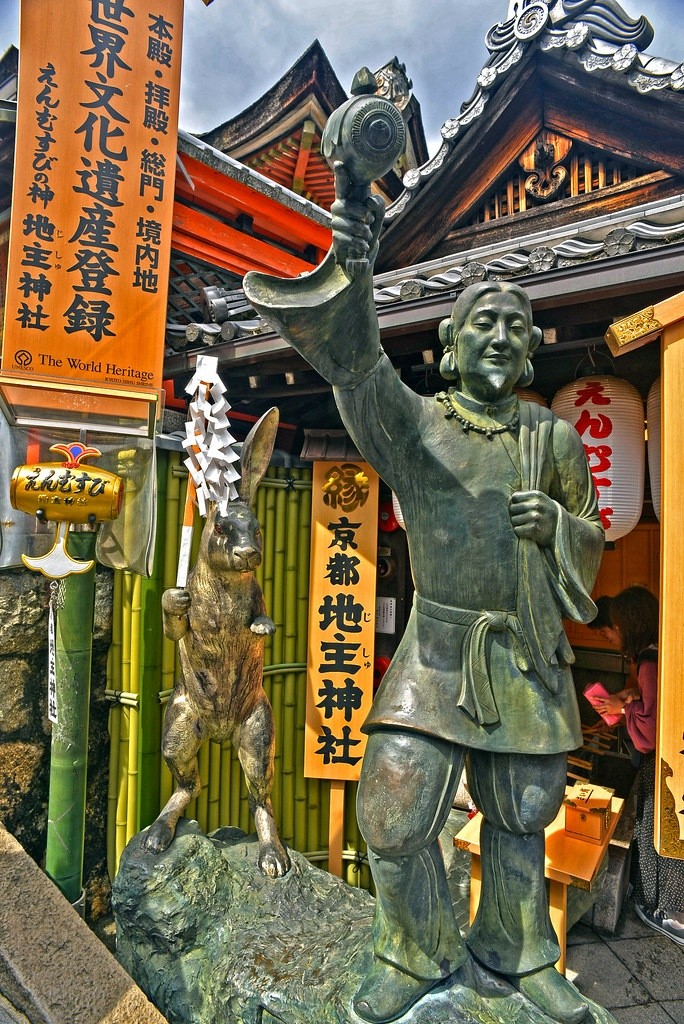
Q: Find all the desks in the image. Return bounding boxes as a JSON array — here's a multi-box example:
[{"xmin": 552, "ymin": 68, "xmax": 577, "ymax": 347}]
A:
[{"xmin": 452, "ymin": 787, "xmax": 626, "ymax": 979}]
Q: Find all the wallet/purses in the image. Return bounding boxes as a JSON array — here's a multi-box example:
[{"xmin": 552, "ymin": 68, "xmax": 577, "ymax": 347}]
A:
[{"xmin": 582, "ymin": 682, "xmax": 622, "ymax": 726}]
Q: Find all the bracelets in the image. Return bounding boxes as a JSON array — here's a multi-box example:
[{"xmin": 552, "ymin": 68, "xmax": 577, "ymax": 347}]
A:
[{"xmin": 621, "ymin": 704, "xmax": 628, "ymax": 714}]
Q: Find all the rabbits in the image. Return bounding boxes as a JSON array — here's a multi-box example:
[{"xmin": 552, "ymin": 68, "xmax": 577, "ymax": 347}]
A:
[{"xmin": 142, "ymin": 395, "xmax": 291, "ymax": 878}]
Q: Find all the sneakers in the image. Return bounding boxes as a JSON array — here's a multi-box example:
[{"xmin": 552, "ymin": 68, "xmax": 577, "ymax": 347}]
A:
[{"xmin": 636, "ymin": 904, "xmax": 684, "ymax": 945}]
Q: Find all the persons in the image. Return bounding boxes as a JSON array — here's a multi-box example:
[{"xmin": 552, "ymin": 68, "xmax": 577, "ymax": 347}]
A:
[
  {"xmin": 242, "ymin": 156, "xmax": 607, "ymax": 1022},
  {"xmin": 585, "ymin": 584, "xmax": 683, "ymax": 949}
]
